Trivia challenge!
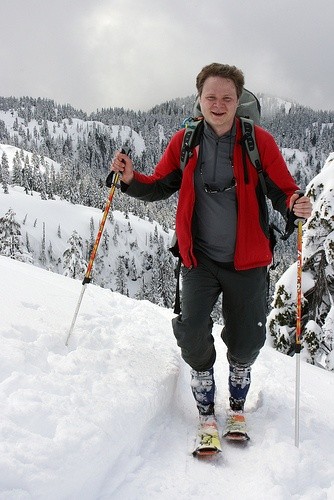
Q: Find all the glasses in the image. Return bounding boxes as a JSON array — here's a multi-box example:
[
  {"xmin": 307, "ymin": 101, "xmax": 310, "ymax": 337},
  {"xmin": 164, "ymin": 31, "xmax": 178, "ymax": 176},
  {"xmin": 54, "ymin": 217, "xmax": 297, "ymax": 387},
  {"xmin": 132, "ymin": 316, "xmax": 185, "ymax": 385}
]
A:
[{"xmin": 200, "ymin": 160, "xmax": 238, "ymax": 194}]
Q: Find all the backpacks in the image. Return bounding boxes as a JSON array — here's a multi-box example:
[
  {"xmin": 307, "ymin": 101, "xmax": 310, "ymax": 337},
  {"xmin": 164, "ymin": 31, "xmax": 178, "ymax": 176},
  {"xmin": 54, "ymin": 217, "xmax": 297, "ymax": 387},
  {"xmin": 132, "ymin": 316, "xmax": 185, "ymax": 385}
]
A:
[{"xmin": 178, "ymin": 83, "xmax": 268, "ymax": 202}]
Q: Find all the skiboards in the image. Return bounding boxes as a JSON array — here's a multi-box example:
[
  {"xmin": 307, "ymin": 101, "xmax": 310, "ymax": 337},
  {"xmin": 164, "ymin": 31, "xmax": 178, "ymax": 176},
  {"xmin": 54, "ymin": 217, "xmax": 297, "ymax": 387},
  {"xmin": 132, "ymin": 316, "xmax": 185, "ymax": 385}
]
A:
[{"xmin": 192, "ymin": 422, "xmax": 250, "ymax": 457}]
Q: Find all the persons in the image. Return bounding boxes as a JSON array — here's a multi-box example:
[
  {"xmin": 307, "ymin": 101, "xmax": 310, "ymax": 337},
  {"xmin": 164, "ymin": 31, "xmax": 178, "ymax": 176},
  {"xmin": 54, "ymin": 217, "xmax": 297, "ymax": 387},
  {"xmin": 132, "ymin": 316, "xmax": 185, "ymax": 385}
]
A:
[{"xmin": 110, "ymin": 63, "xmax": 313, "ymax": 454}]
